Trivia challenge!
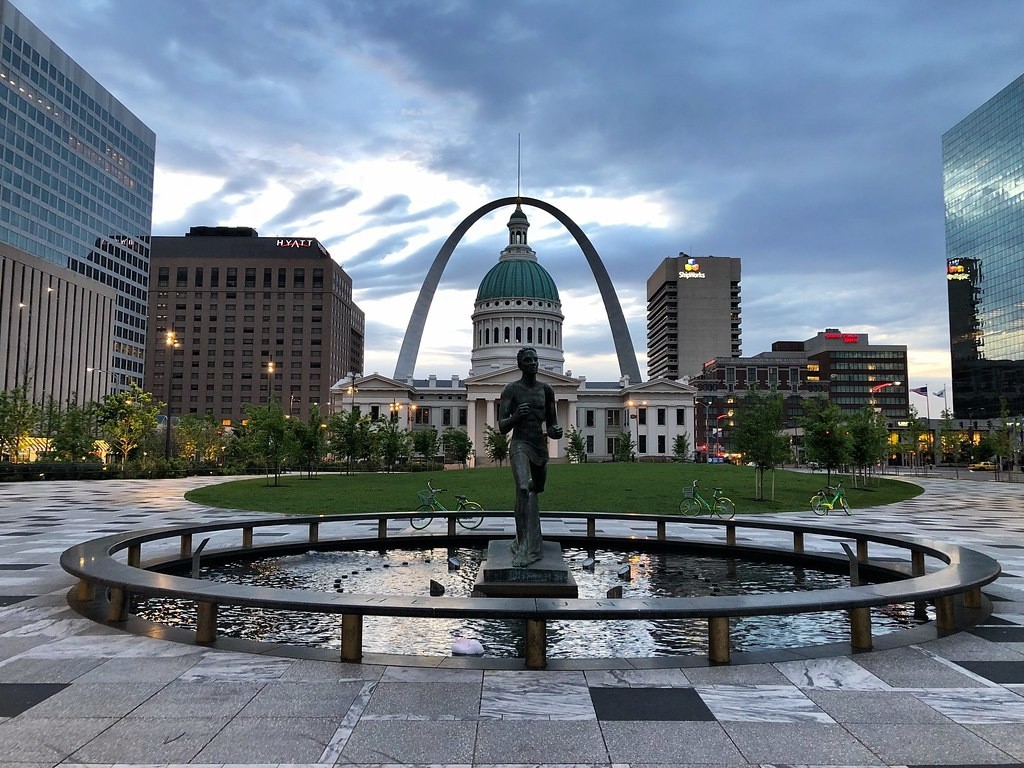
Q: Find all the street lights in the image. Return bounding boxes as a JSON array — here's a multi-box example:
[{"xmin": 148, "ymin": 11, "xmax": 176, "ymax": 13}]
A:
[
  {"xmin": 627, "ymin": 401, "xmax": 648, "ymax": 454},
  {"xmin": 408, "ymin": 404, "xmax": 416, "ymax": 432},
  {"xmin": 164, "ymin": 322, "xmax": 177, "ymax": 465},
  {"xmin": 390, "ymin": 396, "xmax": 402, "ymax": 432},
  {"xmin": 267, "ymin": 354, "xmax": 274, "ymax": 412},
  {"xmin": 347, "ymin": 377, "xmax": 359, "ymax": 421},
  {"xmin": 695, "ymin": 401, "xmax": 713, "ymax": 463},
  {"xmin": 87, "ymin": 367, "xmax": 119, "ymax": 396}
]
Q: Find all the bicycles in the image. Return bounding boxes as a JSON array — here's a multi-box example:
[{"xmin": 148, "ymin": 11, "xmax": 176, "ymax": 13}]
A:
[
  {"xmin": 679, "ymin": 478, "xmax": 736, "ymax": 519},
  {"xmin": 410, "ymin": 479, "xmax": 485, "ymax": 529},
  {"xmin": 808, "ymin": 479, "xmax": 852, "ymax": 516}
]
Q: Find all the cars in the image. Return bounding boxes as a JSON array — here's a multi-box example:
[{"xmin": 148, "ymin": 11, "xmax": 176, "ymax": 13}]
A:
[{"xmin": 968, "ymin": 461, "xmax": 1000, "ymax": 471}]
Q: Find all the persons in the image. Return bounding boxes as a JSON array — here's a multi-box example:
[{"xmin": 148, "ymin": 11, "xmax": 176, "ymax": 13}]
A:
[{"xmin": 498, "ymin": 348, "xmax": 563, "ymax": 565}]
